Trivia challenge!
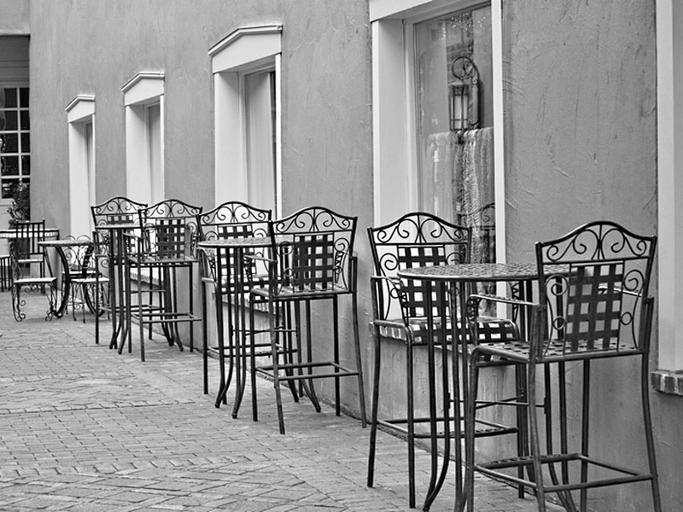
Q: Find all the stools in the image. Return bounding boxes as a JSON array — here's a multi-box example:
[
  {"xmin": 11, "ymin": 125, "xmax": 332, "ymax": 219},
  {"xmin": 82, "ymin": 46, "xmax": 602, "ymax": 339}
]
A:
[
  {"xmin": 1, "ymin": 219, "xmax": 111, "ymax": 323},
  {"xmin": 465, "ymin": 219, "xmax": 661, "ymax": 512},
  {"xmin": 197, "ymin": 201, "xmax": 367, "ymax": 434},
  {"xmin": 90, "ymin": 198, "xmax": 203, "ymax": 363},
  {"xmin": 367, "ymin": 211, "xmax": 526, "ymax": 509}
]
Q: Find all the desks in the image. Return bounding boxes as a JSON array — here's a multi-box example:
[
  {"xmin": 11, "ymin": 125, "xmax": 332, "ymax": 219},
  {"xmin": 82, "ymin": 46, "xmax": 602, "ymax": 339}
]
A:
[{"xmin": 396, "ymin": 264, "xmax": 587, "ymax": 512}]
